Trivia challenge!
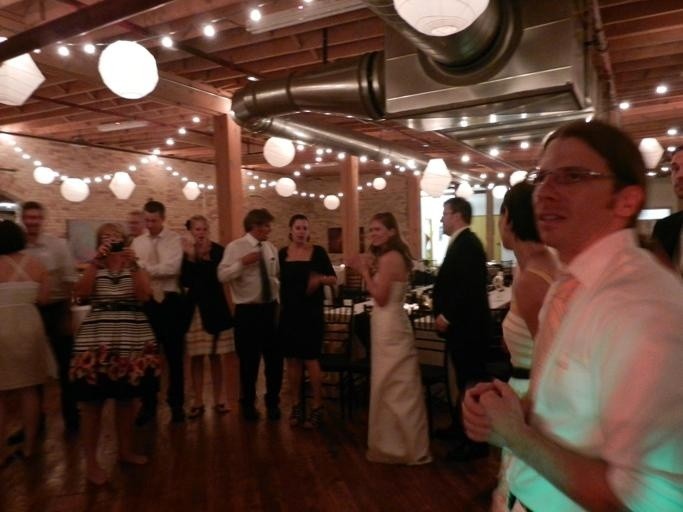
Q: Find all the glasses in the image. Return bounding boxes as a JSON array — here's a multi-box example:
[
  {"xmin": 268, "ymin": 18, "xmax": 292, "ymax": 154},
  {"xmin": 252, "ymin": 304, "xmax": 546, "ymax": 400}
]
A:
[{"xmin": 524, "ymin": 167, "xmax": 614, "ymax": 186}]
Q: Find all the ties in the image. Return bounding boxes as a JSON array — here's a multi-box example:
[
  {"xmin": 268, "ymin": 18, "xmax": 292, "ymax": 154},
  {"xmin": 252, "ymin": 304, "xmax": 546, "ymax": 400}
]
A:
[{"xmin": 258, "ymin": 243, "xmax": 271, "ymax": 300}]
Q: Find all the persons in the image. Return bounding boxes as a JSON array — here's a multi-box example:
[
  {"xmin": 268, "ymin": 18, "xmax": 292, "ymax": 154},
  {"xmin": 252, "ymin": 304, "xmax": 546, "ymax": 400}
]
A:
[
  {"xmin": 276, "ymin": 214, "xmax": 337, "ymax": 429},
  {"xmin": 343, "ymin": 211, "xmax": 431, "ymax": 467},
  {"xmin": 129, "ymin": 202, "xmax": 186, "ymax": 422},
  {"xmin": 0, "ymin": 220, "xmax": 52, "ymax": 467},
  {"xmin": 436, "ymin": 198, "xmax": 488, "ymax": 465},
  {"xmin": 178, "ymin": 212, "xmax": 233, "ymax": 419},
  {"xmin": 495, "ymin": 179, "xmax": 565, "ymax": 512},
  {"xmin": 122, "ymin": 209, "xmax": 147, "ymax": 246},
  {"xmin": 9, "ymin": 200, "xmax": 77, "ymax": 443},
  {"xmin": 651, "ymin": 140, "xmax": 682, "ymax": 280},
  {"xmin": 64, "ymin": 222, "xmax": 162, "ymax": 489},
  {"xmin": 462, "ymin": 123, "xmax": 681, "ymax": 512},
  {"xmin": 216, "ymin": 206, "xmax": 281, "ymax": 427}
]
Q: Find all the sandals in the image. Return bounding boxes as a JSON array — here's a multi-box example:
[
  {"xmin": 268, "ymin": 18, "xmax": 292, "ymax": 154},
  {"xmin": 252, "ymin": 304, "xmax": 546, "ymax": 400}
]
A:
[
  {"xmin": 308, "ymin": 405, "xmax": 324, "ymax": 425},
  {"xmin": 289, "ymin": 402, "xmax": 305, "ymax": 423}
]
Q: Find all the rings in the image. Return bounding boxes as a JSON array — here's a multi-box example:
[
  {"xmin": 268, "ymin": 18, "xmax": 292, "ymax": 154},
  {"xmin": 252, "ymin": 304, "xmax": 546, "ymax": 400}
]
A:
[{"xmin": 102, "ymin": 252, "xmax": 107, "ymax": 256}]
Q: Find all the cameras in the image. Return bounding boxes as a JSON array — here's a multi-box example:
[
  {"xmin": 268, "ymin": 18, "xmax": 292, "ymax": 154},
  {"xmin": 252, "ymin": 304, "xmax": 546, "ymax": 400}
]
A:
[{"xmin": 110, "ymin": 242, "xmax": 124, "ymax": 252}]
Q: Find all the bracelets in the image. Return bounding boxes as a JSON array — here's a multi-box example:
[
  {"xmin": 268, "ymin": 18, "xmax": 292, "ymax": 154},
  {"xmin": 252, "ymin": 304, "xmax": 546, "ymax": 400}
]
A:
[{"xmin": 321, "ymin": 273, "xmax": 331, "ymax": 286}]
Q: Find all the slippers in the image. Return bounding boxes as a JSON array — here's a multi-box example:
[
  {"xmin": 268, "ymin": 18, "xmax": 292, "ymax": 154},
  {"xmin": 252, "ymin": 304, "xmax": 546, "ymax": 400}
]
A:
[
  {"xmin": 211, "ymin": 403, "xmax": 231, "ymax": 413},
  {"xmin": 187, "ymin": 405, "xmax": 205, "ymax": 417}
]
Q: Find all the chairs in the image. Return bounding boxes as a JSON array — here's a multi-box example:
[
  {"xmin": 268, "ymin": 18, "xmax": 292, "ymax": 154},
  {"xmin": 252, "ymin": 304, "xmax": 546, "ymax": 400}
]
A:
[
  {"xmin": 313, "ymin": 306, "xmax": 362, "ymax": 409},
  {"xmin": 404, "ymin": 306, "xmax": 456, "ymax": 423}
]
